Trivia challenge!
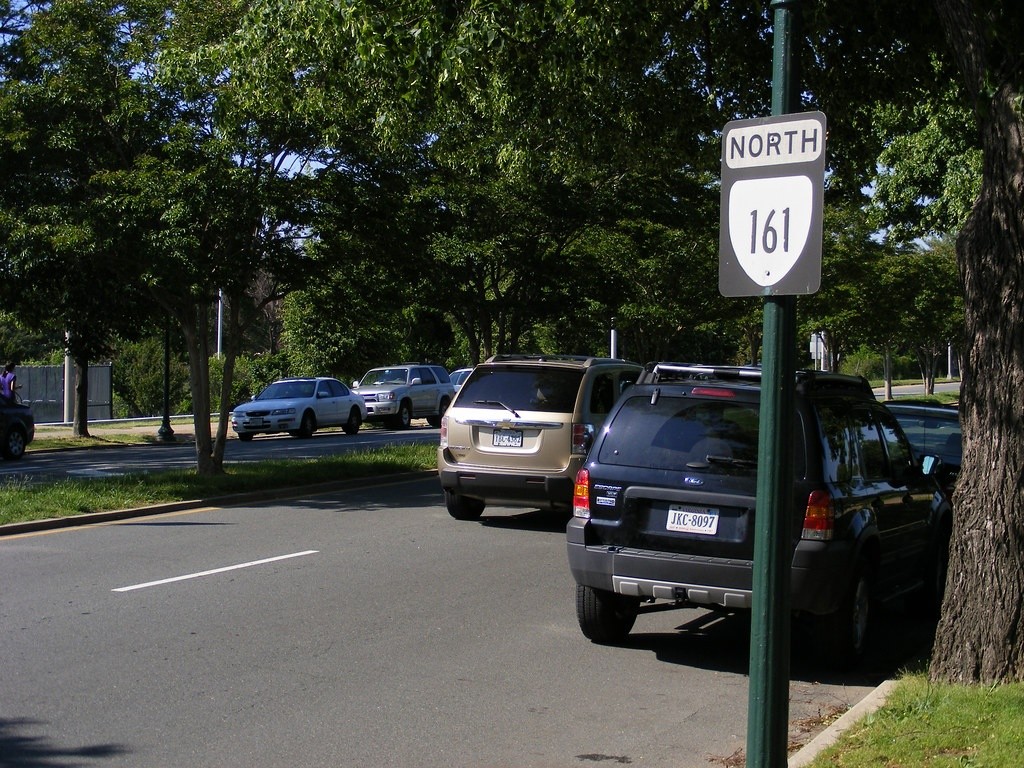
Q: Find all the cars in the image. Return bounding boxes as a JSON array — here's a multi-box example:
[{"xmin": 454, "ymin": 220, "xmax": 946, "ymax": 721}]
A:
[
  {"xmin": 230, "ymin": 377, "xmax": 368, "ymax": 443},
  {"xmin": 0, "ymin": 394, "xmax": 35, "ymax": 460},
  {"xmin": 867, "ymin": 396, "xmax": 964, "ymax": 501},
  {"xmin": 448, "ymin": 367, "xmax": 476, "ymax": 396}
]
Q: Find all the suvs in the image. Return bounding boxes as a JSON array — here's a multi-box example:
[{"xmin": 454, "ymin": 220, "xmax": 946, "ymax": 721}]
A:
[
  {"xmin": 439, "ymin": 351, "xmax": 641, "ymax": 520},
  {"xmin": 350, "ymin": 362, "xmax": 456, "ymax": 430},
  {"xmin": 566, "ymin": 360, "xmax": 951, "ymax": 661}
]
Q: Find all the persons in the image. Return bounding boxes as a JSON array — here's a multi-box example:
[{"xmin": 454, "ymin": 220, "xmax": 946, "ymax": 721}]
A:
[{"xmin": 0, "ymin": 361, "xmax": 23, "ymax": 403}]
